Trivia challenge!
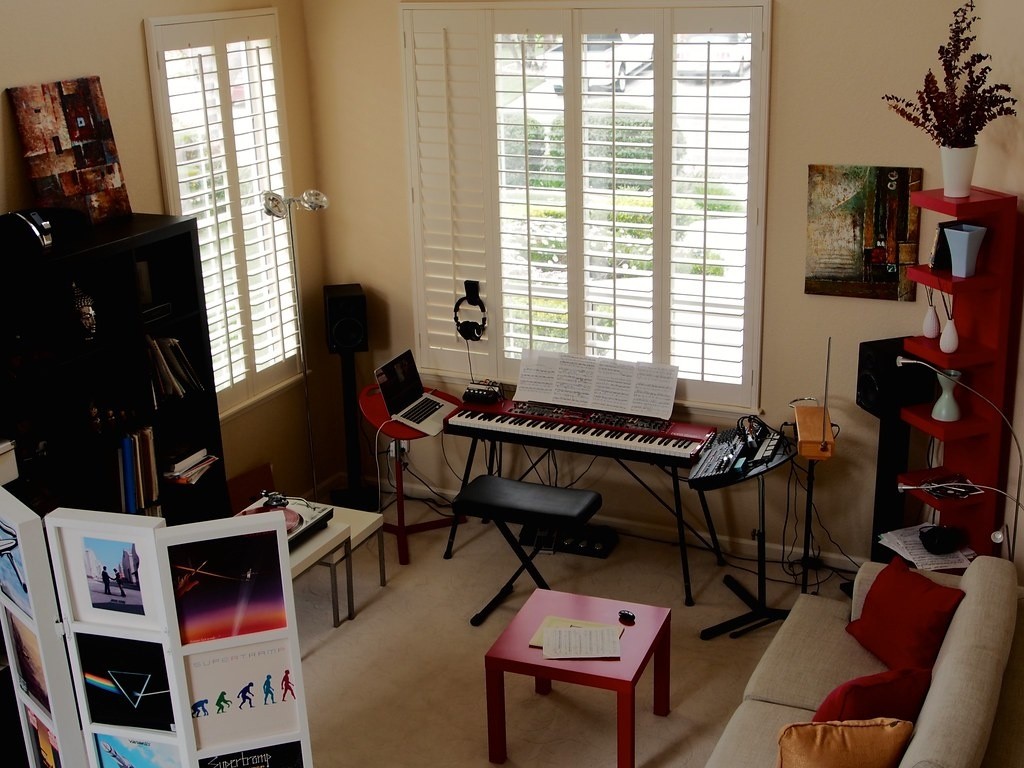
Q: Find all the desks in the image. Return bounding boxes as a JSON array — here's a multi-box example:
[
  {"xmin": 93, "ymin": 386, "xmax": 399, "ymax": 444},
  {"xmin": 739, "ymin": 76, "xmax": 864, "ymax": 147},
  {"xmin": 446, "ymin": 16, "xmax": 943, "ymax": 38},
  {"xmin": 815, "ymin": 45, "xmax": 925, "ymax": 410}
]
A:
[
  {"xmin": 485, "ymin": 588, "xmax": 672, "ymax": 768},
  {"xmin": 699, "ymin": 436, "xmax": 799, "ymax": 641},
  {"xmin": 359, "ymin": 384, "xmax": 466, "ymax": 565},
  {"xmin": 232, "ymin": 494, "xmax": 386, "ymax": 629}
]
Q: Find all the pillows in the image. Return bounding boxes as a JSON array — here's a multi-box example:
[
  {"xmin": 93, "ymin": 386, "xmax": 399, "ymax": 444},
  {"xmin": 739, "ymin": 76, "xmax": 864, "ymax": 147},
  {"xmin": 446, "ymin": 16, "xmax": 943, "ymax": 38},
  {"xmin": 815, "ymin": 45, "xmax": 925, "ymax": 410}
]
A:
[
  {"xmin": 776, "ymin": 716, "xmax": 913, "ymax": 768},
  {"xmin": 810, "ymin": 669, "xmax": 933, "ymax": 724},
  {"xmin": 844, "ymin": 555, "xmax": 966, "ymax": 671}
]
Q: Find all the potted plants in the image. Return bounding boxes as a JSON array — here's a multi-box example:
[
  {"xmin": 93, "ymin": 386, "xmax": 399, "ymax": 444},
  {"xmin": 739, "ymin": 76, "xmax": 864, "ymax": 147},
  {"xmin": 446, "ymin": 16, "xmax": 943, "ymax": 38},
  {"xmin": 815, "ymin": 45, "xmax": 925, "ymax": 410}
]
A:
[{"xmin": 881, "ymin": 0, "xmax": 1017, "ymax": 198}]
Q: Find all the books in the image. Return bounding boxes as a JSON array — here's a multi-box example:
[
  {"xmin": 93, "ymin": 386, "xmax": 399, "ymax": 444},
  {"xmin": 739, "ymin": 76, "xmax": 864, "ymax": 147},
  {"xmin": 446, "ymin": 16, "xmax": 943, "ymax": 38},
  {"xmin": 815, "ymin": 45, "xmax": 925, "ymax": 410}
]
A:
[
  {"xmin": 529, "ymin": 615, "xmax": 625, "ymax": 660},
  {"xmin": 877, "ymin": 522, "xmax": 976, "ymax": 571},
  {"xmin": 117, "ymin": 425, "xmax": 159, "ymax": 513},
  {"xmin": 160, "ymin": 447, "xmax": 219, "ymax": 484},
  {"xmin": 144, "ymin": 331, "xmax": 205, "ymax": 411}
]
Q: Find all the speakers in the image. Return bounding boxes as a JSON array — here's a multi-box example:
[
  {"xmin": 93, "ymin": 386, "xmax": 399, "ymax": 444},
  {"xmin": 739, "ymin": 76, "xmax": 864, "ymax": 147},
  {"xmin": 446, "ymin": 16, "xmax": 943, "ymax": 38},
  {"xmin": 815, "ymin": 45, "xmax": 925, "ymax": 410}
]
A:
[
  {"xmin": 855, "ymin": 335, "xmax": 937, "ymax": 420},
  {"xmin": 323, "ymin": 284, "xmax": 369, "ymax": 354}
]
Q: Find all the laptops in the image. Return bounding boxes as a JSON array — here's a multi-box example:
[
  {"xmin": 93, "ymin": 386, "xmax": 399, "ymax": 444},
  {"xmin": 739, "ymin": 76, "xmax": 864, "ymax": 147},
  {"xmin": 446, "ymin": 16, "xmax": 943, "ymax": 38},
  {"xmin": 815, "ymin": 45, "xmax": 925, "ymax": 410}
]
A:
[{"xmin": 374, "ymin": 348, "xmax": 459, "ymax": 437}]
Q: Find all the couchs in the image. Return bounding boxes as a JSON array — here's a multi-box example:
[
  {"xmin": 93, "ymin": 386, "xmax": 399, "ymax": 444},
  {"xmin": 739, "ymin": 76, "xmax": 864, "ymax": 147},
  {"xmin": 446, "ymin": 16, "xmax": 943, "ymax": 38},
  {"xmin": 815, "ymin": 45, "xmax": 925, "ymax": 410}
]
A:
[{"xmin": 704, "ymin": 556, "xmax": 1018, "ymax": 768}]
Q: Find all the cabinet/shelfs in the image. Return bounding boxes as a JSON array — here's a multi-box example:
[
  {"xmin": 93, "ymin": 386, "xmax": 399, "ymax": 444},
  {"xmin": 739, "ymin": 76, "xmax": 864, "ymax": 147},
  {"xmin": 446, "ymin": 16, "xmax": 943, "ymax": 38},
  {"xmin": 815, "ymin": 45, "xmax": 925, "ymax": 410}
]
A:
[
  {"xmin": 897, "ymin": 186, "xmax": 1017, "ymax": 556},
  {"xmin": 0, "ymin": 211, "xmax": 231, "ymax": 527}
]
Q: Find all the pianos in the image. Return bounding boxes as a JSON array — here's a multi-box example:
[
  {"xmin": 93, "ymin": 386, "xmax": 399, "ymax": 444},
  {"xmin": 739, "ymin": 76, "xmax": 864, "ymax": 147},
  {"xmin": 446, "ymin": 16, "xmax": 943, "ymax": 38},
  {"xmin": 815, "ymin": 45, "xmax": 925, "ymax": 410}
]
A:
[{"xmin": 443, "ymin": 398, "xmax": 725, "ymax": 607}]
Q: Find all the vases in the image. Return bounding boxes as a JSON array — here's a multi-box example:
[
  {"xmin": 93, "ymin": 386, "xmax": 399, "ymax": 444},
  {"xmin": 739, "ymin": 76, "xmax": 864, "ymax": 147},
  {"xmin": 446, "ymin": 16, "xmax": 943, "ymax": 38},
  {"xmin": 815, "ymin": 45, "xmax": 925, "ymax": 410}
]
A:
[
  {"xmin": 944, "ymin": 224, "xmax": 987, "ymax": 278},
  {"xmin": 940, "ymin": 318, "xmax": 959, "ymax": 353},
  {"xmin": 931, "ymin": 370, "xmax": 961, "ymax": 422},
  {"xmin": 923, "ymin": 304, "xmax": 940, "ymax": 339}
]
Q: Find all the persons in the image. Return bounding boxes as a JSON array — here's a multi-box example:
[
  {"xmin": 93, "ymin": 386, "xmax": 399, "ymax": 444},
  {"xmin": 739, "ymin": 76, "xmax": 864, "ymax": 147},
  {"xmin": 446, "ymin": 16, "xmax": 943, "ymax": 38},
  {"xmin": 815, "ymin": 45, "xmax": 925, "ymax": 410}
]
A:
[
  {"xmin": 102, "ymin": 566, "xmax": 113, "ymax": 595},
  {"xmin": 72, "ymin": 282, "xmax": 96, "ymax": 341},
  {"xmin": 113, "ymin": 568, "xmax": 126, "ymax": 597}
]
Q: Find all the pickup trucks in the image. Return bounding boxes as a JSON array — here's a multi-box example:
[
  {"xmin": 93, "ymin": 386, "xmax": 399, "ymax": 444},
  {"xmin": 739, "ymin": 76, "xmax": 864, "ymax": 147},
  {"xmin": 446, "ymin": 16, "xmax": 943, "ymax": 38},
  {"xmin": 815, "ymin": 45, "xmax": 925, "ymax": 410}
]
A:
[{"xmin": 545, "ymin": 34, "xmax": 654, "ymax": 96}]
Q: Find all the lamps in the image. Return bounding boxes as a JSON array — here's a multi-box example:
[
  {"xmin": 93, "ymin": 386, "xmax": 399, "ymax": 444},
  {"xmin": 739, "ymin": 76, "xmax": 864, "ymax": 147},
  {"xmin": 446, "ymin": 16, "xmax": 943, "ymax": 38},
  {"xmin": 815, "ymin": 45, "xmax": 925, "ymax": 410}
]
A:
[{"xmin": 263, "ymin": 190, "xmax": 329, "ymax": 502}]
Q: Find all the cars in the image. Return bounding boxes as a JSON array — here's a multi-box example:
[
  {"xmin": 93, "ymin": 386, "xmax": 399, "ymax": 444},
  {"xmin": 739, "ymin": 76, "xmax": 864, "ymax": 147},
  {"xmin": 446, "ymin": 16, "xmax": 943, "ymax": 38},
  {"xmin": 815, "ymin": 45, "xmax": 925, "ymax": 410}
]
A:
[{"xmin": 676, "ymin": 32, "xmax": 751, "ymax": 82}]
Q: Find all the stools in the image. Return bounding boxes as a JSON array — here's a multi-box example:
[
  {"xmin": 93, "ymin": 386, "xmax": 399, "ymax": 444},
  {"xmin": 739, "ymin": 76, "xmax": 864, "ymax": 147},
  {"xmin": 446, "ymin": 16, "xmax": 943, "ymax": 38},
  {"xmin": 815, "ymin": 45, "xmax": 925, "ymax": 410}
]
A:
[{"xmin": 452, "ymin": 474, "xmax": 603, "ymax": 627}]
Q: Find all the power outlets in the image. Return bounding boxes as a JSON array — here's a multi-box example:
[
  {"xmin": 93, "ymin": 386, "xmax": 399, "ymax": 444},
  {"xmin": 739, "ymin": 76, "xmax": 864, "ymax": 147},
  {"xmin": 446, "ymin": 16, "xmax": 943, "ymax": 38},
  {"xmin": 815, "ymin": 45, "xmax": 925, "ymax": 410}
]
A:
[{"xmin": 389, "ymin": 437, "xmax": 410, "ymax": 458}]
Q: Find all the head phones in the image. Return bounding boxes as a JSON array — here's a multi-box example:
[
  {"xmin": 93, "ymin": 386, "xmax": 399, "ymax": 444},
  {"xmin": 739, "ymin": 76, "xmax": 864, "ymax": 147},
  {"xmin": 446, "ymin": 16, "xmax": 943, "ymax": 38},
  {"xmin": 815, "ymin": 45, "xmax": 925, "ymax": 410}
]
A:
[{"xmin": 454, "ymin": 296, "xmax": 487, "ymax": 341}]
